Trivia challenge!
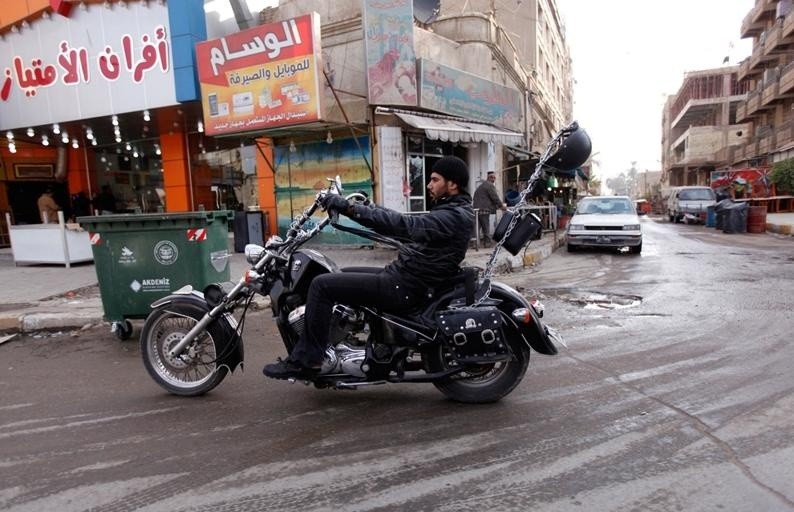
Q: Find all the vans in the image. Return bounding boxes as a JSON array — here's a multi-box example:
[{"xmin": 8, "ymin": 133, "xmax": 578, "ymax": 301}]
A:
[{"xmin": 666, "ymin": 183, "xmax": 719, "ymax": 226}]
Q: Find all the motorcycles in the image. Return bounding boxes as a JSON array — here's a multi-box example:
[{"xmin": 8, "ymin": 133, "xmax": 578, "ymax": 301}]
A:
[{"xmin": 139, "ymin": 120, "xmax": 579, "ymax": 408}]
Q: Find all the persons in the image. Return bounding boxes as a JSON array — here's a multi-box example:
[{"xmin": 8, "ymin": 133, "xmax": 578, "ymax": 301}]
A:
[
  {"xmin": 472, "ymin": 171, "xmax": 506, "ymax": 248},
  {"xmin": 37, "ymin": 185, "xmax": 62, "ymax": 223},
  {"xmin": 262, "ymin": 155, "xmax": 475, "ymax": 380},
  {"xmin": 71, "ymin": 191, "xmax": 92, "ymax": 217},
  {"xmin": 93, "ymin": 185, "xmax": 124, "ymax": 214}
]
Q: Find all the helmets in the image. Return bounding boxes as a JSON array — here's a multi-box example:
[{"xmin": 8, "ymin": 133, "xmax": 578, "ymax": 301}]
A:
[{"xmin": 543, "ymin": 127, "xmax": 592, "ymax": 172}]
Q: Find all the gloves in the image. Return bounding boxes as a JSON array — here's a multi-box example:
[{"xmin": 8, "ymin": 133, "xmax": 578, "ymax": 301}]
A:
[
  {"xmin": 319, "ymin": 193, "xmax": 350, "ymax": 215},
  {"xmin": 355, "ymin": 190, "xmax": 370, "ymax": 207}
]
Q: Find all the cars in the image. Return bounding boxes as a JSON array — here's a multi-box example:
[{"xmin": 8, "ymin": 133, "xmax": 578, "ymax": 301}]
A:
[{"xmin": 564, "ymin": 195, "xmax": 645, "ymax": 257}]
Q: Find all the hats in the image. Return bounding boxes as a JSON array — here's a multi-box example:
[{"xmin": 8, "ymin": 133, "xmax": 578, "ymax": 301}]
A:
[{"xmin": 430, "ymin": 155, "xmax": 469, "ymax": 187}]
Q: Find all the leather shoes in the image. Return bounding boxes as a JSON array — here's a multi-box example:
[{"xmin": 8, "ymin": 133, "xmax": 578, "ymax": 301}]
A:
[{"xmin": 263, "ymin": 360, "xmax": 321, "ymax": 382}]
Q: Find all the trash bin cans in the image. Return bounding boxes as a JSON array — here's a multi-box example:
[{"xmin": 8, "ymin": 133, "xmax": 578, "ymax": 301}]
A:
[{"xmin": 707, "ymin": 206, "xmax": 717, "ymax": 227}]
